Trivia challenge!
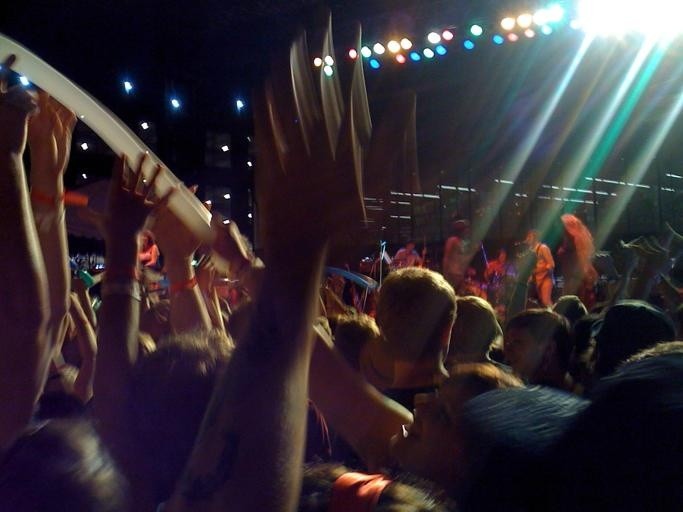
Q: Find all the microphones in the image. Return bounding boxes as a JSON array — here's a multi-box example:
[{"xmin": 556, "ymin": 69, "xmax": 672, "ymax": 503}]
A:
[{"xmin": 381, "ymin": 241, "xmax": 387, "ymax": 246}]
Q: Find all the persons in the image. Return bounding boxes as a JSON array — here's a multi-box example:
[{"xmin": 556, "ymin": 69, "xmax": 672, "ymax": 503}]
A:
[{"xmin": 0, "ymin": 48, "xmax": 682, "ymax": 511}]
